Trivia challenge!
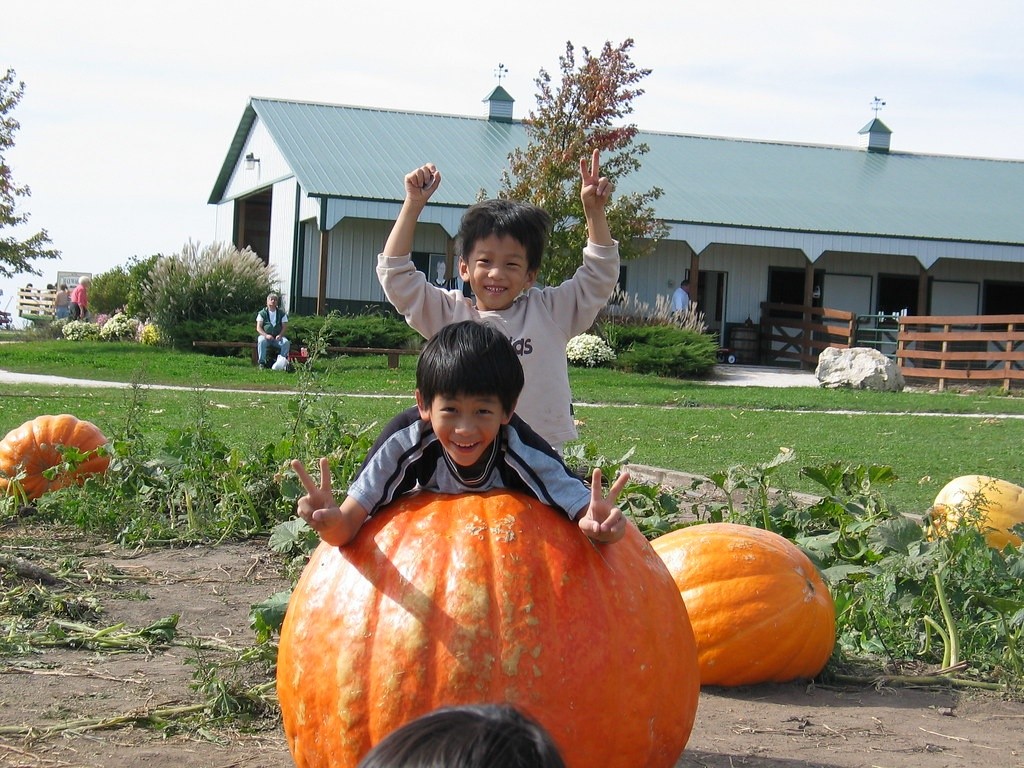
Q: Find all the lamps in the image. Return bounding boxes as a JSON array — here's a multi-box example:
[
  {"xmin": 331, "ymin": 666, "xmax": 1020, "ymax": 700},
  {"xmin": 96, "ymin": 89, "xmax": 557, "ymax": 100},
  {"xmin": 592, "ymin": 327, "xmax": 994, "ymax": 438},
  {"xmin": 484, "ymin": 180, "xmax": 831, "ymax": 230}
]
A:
[{"xmin": 245, "ymin": 153, "xmax": 260, "ymax": 170}]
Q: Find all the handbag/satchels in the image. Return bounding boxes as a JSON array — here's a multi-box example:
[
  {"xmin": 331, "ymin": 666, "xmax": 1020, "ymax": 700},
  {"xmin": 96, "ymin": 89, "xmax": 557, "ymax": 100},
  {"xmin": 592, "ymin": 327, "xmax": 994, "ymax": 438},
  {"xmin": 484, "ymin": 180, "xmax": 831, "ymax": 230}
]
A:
[{"xmin": 272, "ymin": 354, "xmax": 289, "ymax": 372}]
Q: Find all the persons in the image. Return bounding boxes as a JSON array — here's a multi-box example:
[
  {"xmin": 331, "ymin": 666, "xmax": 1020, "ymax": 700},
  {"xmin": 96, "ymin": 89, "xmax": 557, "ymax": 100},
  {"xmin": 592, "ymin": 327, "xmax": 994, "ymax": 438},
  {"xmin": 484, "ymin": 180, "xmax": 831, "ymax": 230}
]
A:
[
  {"xmin": 291, "ymin": 320, "xmax": 630, "ymax": 547},
  {"xmin": 356, "ymin": 703, "xmax": 567, "ymax": 768},
  {"xmin": 670, "ymin": 279, "xmax": 689, "ymax": 321},
  {"xmin": 256, "ymin": 293, "xmax": 290, "ymax": 369},
  {"xmin": 376, "ymin": 148, "xmax": 620, "ymax": 456},
  {"xmin": 25, "ymin": 283, "xmax": 35, "ymax": 314},
  {"xmin": 55, "ymin": 276, "xmax": 89, "ymax": 321}
]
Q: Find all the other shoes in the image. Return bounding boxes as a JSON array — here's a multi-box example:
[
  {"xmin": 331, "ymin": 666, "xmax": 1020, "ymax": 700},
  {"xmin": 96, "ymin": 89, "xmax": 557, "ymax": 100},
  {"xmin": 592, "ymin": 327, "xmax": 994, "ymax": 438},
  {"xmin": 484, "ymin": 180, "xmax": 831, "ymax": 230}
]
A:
[{"xmin": 259, "ymin": 364, "xmax": 265, "ymax": 370}]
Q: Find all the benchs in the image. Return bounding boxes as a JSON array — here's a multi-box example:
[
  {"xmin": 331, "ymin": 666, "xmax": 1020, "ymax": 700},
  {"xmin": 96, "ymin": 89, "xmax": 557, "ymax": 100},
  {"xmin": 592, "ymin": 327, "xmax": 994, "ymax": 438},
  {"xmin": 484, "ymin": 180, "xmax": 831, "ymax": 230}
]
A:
[{"xmin": 191, "ymin": 340, "xmax": 420, "ymax": 369}]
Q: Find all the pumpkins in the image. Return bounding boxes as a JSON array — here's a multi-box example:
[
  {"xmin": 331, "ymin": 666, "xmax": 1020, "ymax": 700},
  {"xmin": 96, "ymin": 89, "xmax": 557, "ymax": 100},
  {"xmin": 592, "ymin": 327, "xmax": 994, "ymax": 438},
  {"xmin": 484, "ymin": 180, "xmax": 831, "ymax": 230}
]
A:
[
  {"xmin": 645, "ymin": 523, "xmax": 834, "ymax": 686},
  {"xmin": 0, "ymin": 413, "xmax": 111, "ymax": 501},
  {"xmin": 276, "ymin": 484, "xmax": 699, "ymax": 768},
  {"xmin": 926, "ymin": 476, "xmax": 1024, "ymax": 550}
]
